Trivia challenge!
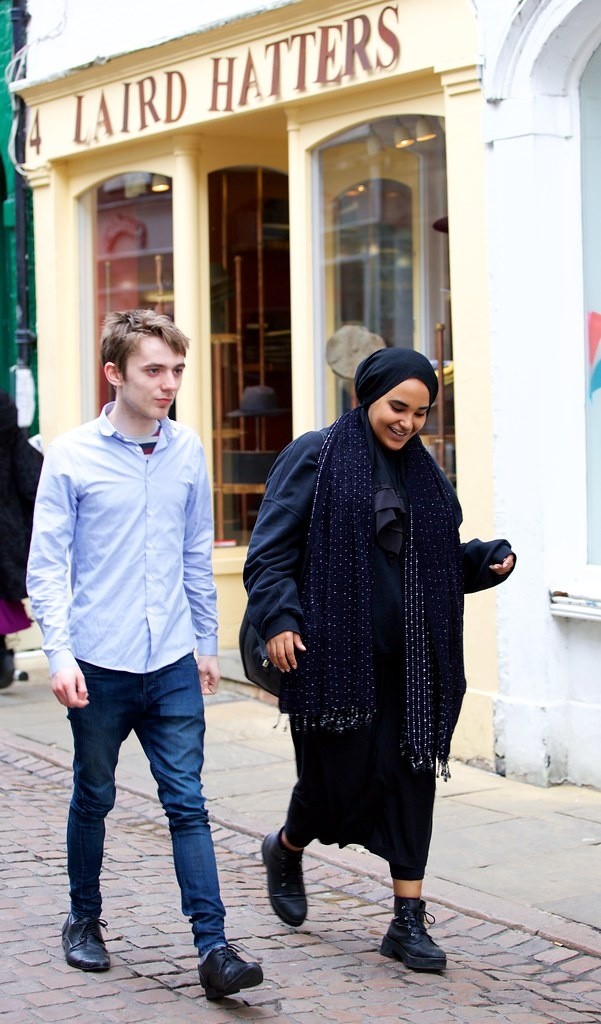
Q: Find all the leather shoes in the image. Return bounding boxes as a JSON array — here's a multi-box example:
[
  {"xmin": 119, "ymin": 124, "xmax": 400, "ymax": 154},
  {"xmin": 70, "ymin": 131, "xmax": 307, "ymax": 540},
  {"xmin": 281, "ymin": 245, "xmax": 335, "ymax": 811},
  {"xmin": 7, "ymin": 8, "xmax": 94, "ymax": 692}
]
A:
[
  {"xmin": 195, "ymin": 942, "xmax": 265, "ymax": 1001},
  {"xmin": 60, "ymin": 912, "xmax": 112, "ymax": 971}
]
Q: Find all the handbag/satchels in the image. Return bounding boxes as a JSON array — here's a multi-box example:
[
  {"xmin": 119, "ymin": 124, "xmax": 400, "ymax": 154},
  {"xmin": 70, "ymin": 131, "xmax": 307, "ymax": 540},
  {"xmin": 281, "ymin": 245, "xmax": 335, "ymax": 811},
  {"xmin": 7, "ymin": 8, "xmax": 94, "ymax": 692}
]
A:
[{"xmin": 238, "ymin": 605, "xmax": 284, "ymax": 697}]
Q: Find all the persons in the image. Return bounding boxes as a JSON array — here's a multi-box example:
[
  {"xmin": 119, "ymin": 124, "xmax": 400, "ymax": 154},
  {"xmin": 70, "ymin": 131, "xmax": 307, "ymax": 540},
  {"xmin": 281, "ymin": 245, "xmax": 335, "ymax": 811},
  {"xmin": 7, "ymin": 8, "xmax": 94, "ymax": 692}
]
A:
[
  {"xmin": 0, "ymin": 388, "xmax": 45, "ymax": 688},
  {"xmin": 26, "ymin": 310, "xmax": 263, "ymax": 1002},
  {"xmin": 244, "ymin": 348, "xmax": 516, "ymax": 969}
]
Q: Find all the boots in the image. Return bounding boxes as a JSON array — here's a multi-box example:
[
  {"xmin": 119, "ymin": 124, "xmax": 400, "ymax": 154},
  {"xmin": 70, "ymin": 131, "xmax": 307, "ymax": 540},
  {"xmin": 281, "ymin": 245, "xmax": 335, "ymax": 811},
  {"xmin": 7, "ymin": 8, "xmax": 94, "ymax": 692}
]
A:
[
  {"xmin": 260, "ymin": 826, "xmax": 309, "ymax": 927},
  {"xmin": 380, "ymin": 896, "xmax": 448, "ymax": 970}
]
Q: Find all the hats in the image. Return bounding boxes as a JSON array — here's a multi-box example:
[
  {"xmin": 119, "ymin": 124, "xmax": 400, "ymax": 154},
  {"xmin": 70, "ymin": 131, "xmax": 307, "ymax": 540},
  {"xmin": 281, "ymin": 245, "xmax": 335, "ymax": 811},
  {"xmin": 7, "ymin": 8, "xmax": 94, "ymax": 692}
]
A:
[
  {"xmin": 352, "ymin": 345, "xmax": 439, "ymax": 418},
  {"xmin": 225, "ymin": 385, "xmax": 290, "ymax": 417},
  {"xmin": 326, "ymin": 323, "xmax": 387, "ymax": 377}
]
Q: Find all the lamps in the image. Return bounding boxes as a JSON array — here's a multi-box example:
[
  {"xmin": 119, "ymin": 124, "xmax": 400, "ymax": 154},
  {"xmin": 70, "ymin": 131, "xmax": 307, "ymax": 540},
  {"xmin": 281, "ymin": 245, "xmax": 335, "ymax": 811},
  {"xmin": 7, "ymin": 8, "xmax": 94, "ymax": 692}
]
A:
[
  {"xmin": 365, "ymin": 114, "xmax": 437, "ymax": 156},
  {"xmin": 150, "ymin": 175, "xmax": 171, "ymax": 195}
]
